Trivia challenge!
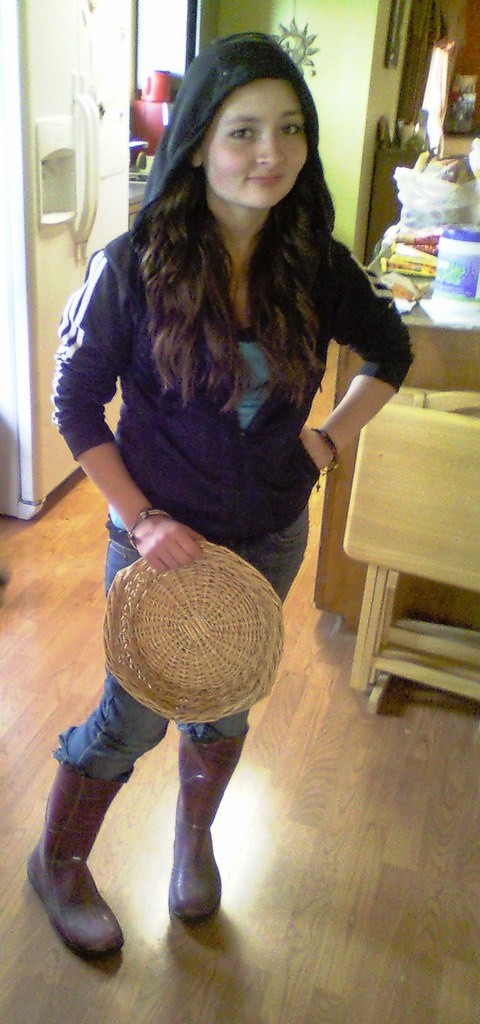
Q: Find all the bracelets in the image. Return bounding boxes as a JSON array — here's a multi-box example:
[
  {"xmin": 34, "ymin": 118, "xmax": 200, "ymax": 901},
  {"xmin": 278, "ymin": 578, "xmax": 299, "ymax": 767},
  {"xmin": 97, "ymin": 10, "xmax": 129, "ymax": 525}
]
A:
[
  {"xmin": 124, "ymin": 508, "xmax": 172, "ymax": 561},
  {"xmin": 314, "ymin": 426, "xmax": 340, "ymax": 491}
]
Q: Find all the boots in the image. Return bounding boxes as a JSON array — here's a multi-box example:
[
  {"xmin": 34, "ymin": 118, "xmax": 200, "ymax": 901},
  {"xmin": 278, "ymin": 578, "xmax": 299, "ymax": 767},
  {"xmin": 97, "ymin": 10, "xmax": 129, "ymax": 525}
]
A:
[
  {"xmin": 26, "ymin": 763, "xmax": 125, "ymax": 962},
  {"xmin": 169, "ymin": 725, "xmax": 249, "ymax": 922}
]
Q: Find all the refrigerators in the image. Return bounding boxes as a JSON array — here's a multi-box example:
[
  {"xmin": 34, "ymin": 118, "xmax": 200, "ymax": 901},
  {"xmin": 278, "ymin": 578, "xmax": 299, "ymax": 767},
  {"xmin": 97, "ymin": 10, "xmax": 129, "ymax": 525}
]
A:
[{"xmin": 0, "ymin": 0, "xmax": 129, "ymax": 521}]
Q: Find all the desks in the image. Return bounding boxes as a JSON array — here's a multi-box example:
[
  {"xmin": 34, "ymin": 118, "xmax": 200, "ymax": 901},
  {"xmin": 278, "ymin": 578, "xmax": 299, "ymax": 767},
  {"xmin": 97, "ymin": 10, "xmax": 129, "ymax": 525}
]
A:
[{"xmin": 343, "ymin": 386, "xmax": 479, "ymax": 716}]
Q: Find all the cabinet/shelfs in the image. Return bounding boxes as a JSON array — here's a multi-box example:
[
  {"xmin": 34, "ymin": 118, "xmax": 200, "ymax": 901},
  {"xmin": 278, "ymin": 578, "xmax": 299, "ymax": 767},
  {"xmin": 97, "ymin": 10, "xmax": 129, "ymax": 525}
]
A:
[{"xmin": 313, "ymin": 241, "xmax": 480, "ymax": 642}]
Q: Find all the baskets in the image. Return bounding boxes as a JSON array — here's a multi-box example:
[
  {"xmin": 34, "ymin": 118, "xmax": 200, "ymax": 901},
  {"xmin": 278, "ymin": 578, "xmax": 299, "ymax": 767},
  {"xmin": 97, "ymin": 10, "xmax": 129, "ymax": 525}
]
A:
[{"xmin": 103, "ymin": 539, "xmax": 284, "ymax": 723}]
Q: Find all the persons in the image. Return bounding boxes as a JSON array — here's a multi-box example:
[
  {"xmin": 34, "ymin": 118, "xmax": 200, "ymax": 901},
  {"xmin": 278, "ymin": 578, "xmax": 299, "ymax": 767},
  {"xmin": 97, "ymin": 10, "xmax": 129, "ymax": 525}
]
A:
[{"xmin": 26, "ymin": 30, "xmax": 413, "ymax": 961}]
